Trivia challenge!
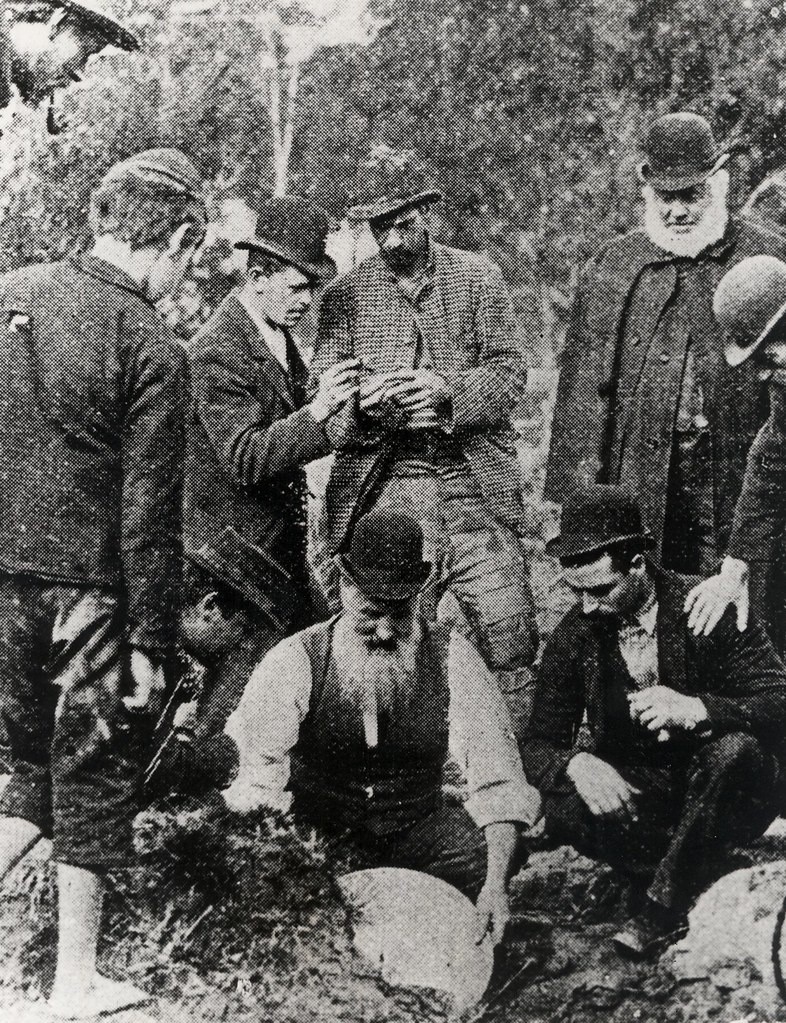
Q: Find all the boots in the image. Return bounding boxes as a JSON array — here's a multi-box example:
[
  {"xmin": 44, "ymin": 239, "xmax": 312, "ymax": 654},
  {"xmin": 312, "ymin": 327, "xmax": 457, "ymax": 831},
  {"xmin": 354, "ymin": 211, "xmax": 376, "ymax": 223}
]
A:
[{"xmin": 46, "ymin": 861, "xmax": 157, "ymax": 1022}]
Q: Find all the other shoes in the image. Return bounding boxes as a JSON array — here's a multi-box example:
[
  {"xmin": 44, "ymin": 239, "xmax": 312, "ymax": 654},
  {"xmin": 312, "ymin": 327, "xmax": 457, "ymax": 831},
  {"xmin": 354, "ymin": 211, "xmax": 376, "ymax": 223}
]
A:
[{"xmin": 609, "ymin": 901, "xmax": 675, "ymax": 961}]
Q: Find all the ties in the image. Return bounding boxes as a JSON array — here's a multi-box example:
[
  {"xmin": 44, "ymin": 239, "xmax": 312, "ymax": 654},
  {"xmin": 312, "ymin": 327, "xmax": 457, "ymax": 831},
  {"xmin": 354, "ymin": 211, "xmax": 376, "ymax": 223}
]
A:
[{"xmin": 265, "ymin": 316, "xmax": 309, "ymax": 414}]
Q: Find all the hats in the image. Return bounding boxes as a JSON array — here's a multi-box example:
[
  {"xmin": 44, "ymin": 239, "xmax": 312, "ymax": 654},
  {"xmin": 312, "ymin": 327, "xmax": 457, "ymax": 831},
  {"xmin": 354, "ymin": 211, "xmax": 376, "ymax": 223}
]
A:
[
  {"xmin": 104, "ymin": 148, "xmax": 209, "ymax": 229},
  {"xmin": 635, "ymin": 112, "xmax": 733, "ymax": 191},
  {"xmin": 331, "ymin": 509, "xmax": 432, "ymax": 601},
  {"xmin": 48, "ymin": 1, "xmax": 143, "ymax": 51},
  {"xmin": 183, "ymin": 525, "xmax": 295, "ymax": 634},
  {"xmin": 543, "ymin": 484, "xmax": 659, "ymax": 559},
  {"xmin": 231, "ymin": 193, "xmax": 340, "ymax": 280},
  {"xmin": 713, "ymin": 253, "xmax": 786, "ymax": 368},
  {"xmin": 346, "ymin": 143, "xmax": 442, "ymax": 222}
]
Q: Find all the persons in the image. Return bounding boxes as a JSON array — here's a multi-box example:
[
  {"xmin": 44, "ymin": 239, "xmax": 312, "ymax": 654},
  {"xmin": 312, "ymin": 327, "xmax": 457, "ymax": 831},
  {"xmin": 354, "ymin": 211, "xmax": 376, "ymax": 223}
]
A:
[
  {"xmin": 184, "ymin": 194, "xmax": 362, "ymax": 633},
  {"xmin": 0, "ymin": 0, "xmax": 146, "ymax": 113},
  {"xmin": 1, "ymin": 147, "xmax": 213, "ymax": 1019},
  {"xmin": 307, "ymin": 153, "xmax": 546, "ymax": 737},
  {"xmin": 543, "ymin": 113, "xmax": 786, "ymax": 612},
  {"xmin": 521, "ymin": 483, "xmax": 786, "ymax": 954},
  {"xmin": 217, "ymin": 514, "xmax": 543, "ymax": 950},
  {"xmin": 682, "ymin": 255, "xmax": 786, "ymax": 635},
  {"xmin": 129, "ymin": 528, "xmax": 292, "ymax": 812}
]
